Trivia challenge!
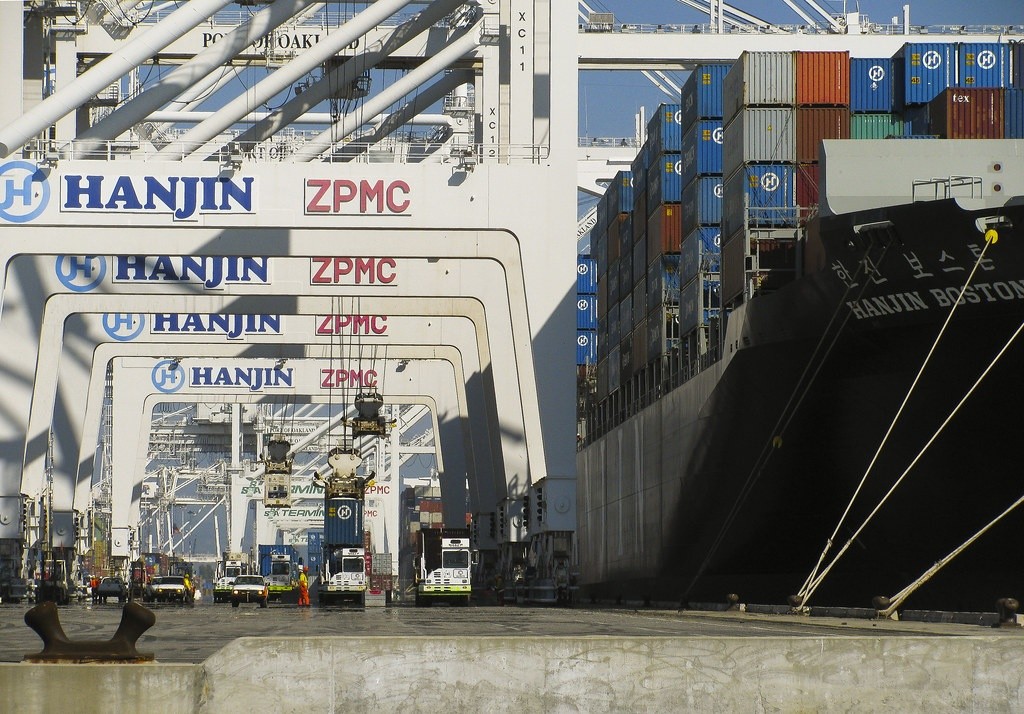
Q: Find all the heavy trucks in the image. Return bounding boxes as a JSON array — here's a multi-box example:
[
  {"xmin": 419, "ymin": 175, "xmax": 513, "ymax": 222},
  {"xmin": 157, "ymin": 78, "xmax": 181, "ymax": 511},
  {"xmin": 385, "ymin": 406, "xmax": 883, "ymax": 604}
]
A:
[
  {"xmin": 315, "ymin": 547, "xmax": 366, "ymax": 607},
  {"xmin": 414, "ymin": 533, "xmax": 479, "ymax": 608},
  {"xmin": 262, "ymin": 554, "xmax": 299, "ymax": 603},
  {"xmin": 212, "ymin": 552, "xmax": 258, "ymax": 603}
]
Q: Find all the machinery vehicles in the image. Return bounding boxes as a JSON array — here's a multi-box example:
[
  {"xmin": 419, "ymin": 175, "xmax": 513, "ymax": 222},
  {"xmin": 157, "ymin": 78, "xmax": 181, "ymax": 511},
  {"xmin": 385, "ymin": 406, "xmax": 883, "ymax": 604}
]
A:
[
  {"xmin": 30, "ymin": 551, "xmax": 70, "ymax": 605},
  {"xmin": 128, "ymin": 561, "xmax": 147, "ymax": 603}
]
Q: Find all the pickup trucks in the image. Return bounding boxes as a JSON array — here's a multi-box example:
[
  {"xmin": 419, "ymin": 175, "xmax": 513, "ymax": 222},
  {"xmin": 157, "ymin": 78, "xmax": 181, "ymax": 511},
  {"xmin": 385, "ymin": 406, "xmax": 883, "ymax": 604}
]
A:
[{"xmin": 97, "ymin": 576, "xmax": 128, "ymax": 602}]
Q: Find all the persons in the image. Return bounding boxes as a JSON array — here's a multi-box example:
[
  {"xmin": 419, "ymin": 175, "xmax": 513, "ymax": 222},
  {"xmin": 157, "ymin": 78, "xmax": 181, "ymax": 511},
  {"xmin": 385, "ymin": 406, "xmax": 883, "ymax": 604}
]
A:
[
  {"xmin": 299, "ymin": 567, "xmax": 310, "ymax": 605},
  {"xmin": 184, "ymin": 574, "xmax": 196, "ymax": 603},
  {"xmin": 494, "ymin": 574, "xmax": 507, "ymax": 606},
  {"xmin": 90, "ymin": 575, "xmax": 101, "ymax": 604},
  {"xmin": 553, "ymin": 560, "xmax": 568, "ymax": 607}
]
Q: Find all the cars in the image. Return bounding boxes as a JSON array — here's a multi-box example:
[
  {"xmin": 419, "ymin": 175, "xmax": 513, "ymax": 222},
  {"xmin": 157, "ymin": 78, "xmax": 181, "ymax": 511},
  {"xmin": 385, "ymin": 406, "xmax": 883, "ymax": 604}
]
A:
[
  {"xmin": 147, "ymin": 576, "xmax": 186, "ymax": 603},
  {"xmin": 227, "ymin": 574, "xmax": 270, "ymax": 608}
]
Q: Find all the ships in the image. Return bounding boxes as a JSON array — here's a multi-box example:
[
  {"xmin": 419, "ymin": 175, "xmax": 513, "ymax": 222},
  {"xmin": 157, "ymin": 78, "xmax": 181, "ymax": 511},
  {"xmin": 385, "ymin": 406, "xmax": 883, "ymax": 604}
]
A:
[{"xmin": 577, "ymin": 139, "xmax": 1024, "ymax": 630}]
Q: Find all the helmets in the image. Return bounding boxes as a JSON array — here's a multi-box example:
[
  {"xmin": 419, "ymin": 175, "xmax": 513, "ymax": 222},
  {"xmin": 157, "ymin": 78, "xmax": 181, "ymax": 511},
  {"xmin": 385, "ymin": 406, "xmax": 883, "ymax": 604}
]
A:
[
  {"xmin": 92, "ymin": 575, "xmax": 96, "ymax": 580},
  {"xmin": 303, "ymin": 566, "xmax": 309, "ymax": 571},
  {"xmin": 185, "ymin": 574, "xmax": 189, "ymax": 577}
]
